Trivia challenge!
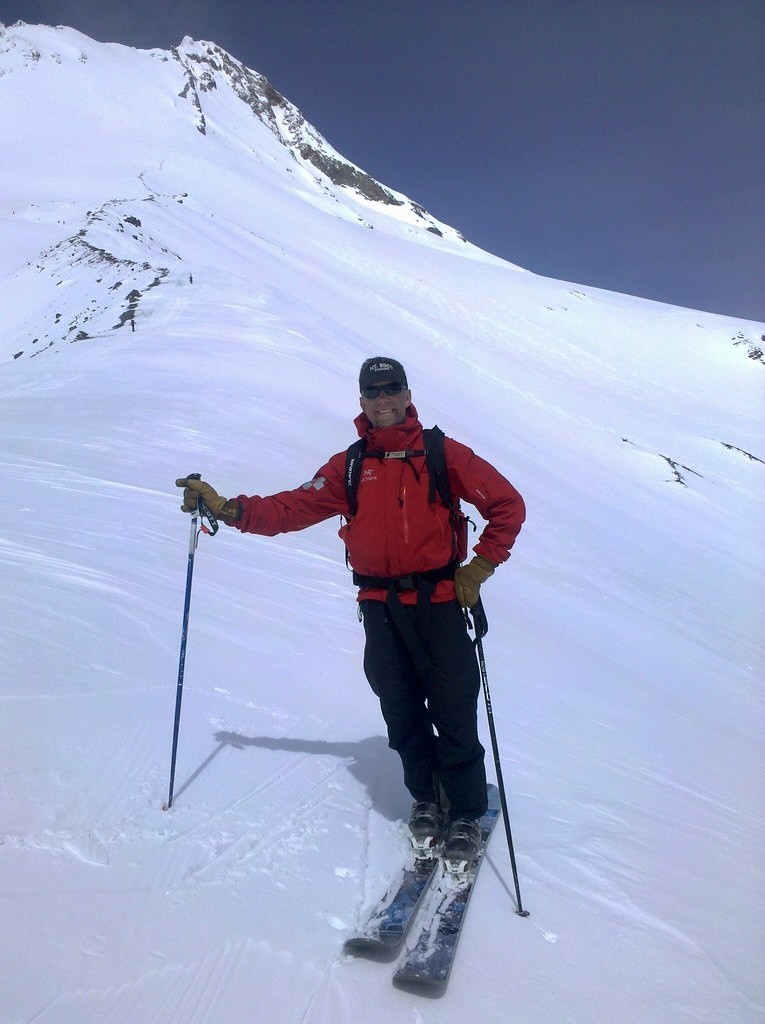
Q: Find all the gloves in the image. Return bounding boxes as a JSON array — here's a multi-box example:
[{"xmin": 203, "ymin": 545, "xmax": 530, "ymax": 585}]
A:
[
  {"xmin": 176, "ymin": 478, "xmax": 227, "ymax": 521},
  {"xmin": 454, "ymin": 556, "xmax": 495, "ymax": 608}
]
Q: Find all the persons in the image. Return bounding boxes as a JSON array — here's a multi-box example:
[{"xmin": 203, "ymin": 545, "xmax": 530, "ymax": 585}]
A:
[{"xmin": 176, "ymin": 357, "xmax": 526, "ymax": 858}]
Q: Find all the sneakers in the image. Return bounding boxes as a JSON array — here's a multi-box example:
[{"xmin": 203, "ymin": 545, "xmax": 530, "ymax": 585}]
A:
[
  {"xmin": 409, "ymin": 803, "xmax": 443, "ymax": 860},
  {"xmin": 442, "ymin": 816, "xmax": 482, "ymax": 873}
]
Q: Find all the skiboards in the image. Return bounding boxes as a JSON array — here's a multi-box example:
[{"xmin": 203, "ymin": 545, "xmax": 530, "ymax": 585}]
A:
[{"xmin": 342, "ymin": 780, "xmax": 504, "ymax": 990}]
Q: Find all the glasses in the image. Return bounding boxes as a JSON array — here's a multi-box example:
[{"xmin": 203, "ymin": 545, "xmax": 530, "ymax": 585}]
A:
[{"xmin": 361, "ymin": 382, "xmax": 408, "ymax": 399}]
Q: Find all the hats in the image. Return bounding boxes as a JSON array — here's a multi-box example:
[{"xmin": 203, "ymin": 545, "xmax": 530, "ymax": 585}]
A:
[{"xmin": 359, "ymin": 357, "xmax": 408, "ymax": 392}]
